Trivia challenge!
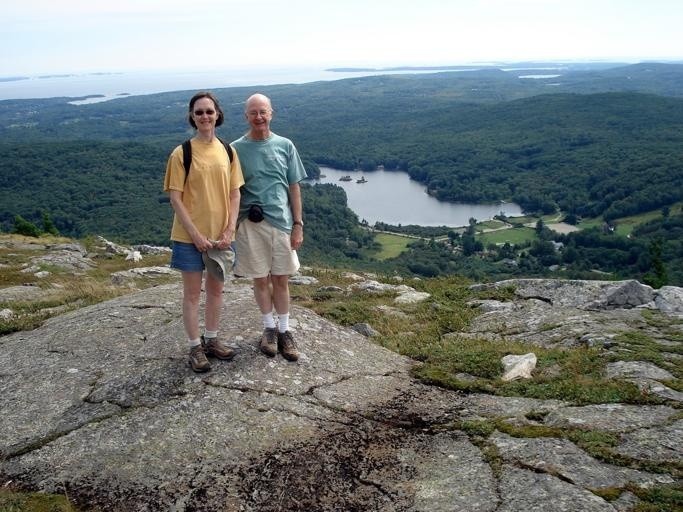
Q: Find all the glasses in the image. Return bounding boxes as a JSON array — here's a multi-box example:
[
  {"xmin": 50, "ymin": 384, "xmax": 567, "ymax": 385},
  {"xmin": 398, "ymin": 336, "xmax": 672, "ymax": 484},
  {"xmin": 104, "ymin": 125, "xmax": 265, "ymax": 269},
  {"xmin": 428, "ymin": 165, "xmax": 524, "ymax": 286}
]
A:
[{"xmin": 195, "ymin": 110, "xmax": 214, "ymax": 115}]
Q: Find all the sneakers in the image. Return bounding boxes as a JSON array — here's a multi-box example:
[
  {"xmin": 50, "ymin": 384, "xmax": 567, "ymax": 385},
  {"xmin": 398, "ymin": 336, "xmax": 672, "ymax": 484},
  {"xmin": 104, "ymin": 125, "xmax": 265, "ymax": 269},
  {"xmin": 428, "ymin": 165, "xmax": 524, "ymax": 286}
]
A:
[
  {"xmin": 260, "ymin": 324, "xmax": 299, "ymax": 361},
  {"xmin": 188, "ymin": 335, "xmax": 234, "ymax": 372}
]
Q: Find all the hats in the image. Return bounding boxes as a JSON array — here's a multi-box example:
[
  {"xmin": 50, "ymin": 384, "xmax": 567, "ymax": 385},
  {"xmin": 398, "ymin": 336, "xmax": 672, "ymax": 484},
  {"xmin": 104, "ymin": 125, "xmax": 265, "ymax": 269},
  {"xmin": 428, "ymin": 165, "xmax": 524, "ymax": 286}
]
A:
[{"xmin": 202, "ymin": 239, "xmax": 236, "ymax": 283}]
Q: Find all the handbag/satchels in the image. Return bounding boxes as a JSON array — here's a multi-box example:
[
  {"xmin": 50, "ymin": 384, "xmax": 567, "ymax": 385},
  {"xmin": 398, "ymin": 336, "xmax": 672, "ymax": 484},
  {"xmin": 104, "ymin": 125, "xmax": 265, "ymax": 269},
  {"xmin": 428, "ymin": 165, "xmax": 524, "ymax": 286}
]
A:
[{"xmin": 248, "ymin": 203, "xmax": 265, "ymax": 222}]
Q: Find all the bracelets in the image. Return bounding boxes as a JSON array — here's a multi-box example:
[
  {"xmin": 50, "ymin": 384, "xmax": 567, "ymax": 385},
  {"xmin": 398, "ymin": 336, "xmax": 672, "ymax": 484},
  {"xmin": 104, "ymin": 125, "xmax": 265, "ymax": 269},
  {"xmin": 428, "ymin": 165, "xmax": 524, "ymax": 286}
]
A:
[{"xmin": 291, "ymin": 220, "xmax": 304, "ymax": 227}]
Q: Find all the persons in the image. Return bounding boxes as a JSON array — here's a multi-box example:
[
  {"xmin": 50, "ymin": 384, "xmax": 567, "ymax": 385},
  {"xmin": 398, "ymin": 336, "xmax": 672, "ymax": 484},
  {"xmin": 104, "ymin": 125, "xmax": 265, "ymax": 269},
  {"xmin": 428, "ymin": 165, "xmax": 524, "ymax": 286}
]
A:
[
  {"xmin": 228, "ymin": 93, "xmax": 309, "ymax": 365},
  {"xmin": 161, "ymin": 90, "xmax": 246, "ymax": 373}
]
